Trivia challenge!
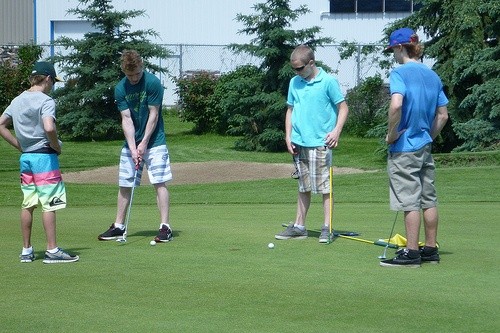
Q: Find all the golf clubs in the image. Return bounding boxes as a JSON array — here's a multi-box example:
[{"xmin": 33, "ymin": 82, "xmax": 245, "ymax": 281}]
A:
[
  {"xmin": 377, "ymin": 211, "xmax": 399, "ymax": 259},
  {"xmin": 116, "ymin": 157, "xmax": 140, "ymax": 244},
  {"xmin": 324, "ymin": 148, "xmax": 334, "ymax": 244}
]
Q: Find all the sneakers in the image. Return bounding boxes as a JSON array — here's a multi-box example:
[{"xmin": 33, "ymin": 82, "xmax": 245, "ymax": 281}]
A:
[
  {"xmin": 273, "ymin": 221, "xmax": 308, "ymax": 240},
  {"xmin": 18, "ymin": 248, "xmax": 38, "ymax": 263},
  {"xmin": 418, "ymin": 241, "xmax": 441, "ymax": 264},
  {"xmin": 97, "ymin": 222, "xmax": 127, "ymax": 241},
  {"xmin": 379, "ymin": 249, "xmax": 423, "ymax": 268},
  {"xmin": 318, "ymin": 225, "xmax": 334, "ymax": 243},
  {"xmin": 42, "ymin": 247, "xmax": 80, "ymax": 264},
  {"xmin": 152, "ymin": 224, "xmax": 174, "ymax": 243}
]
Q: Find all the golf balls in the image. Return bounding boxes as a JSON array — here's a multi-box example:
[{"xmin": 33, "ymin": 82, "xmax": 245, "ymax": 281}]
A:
[
  {"xmin": 268, "ymin": 243, "xmax": 274, "ymax": 248},
  {"xmin": 150, "ymin": 241, "xmax": 156, "ymax": 245}
]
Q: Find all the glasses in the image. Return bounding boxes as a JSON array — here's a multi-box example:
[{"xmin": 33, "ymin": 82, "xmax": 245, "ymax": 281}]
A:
[{"xmin": 291, "ymin": 61, "xmax": 310, "ymax": 71}]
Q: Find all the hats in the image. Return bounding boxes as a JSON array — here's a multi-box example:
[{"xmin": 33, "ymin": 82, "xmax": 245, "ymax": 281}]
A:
[
  {"xmin": 31, "ymin": 61, "xmax": 62, "ymax": 82},
  {"xmin": 381, "ymin": 27, "xmax": 417, "ymax": 53}
]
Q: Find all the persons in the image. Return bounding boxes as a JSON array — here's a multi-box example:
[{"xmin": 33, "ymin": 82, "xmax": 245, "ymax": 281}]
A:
[
  {"xmin": 98, "ymin": 49, "xmax": 173, "ymax": 243},
  {"xmin": 379, "ymin": 28, "xmax": 450, "ymax": 266},
  {"xmin": 274, "ymin": 45, "xmax": 349, "ymax": 242},
  {"xmin": 0, "ymin": 62, "xmax": 79, "ymax": 264}
]
